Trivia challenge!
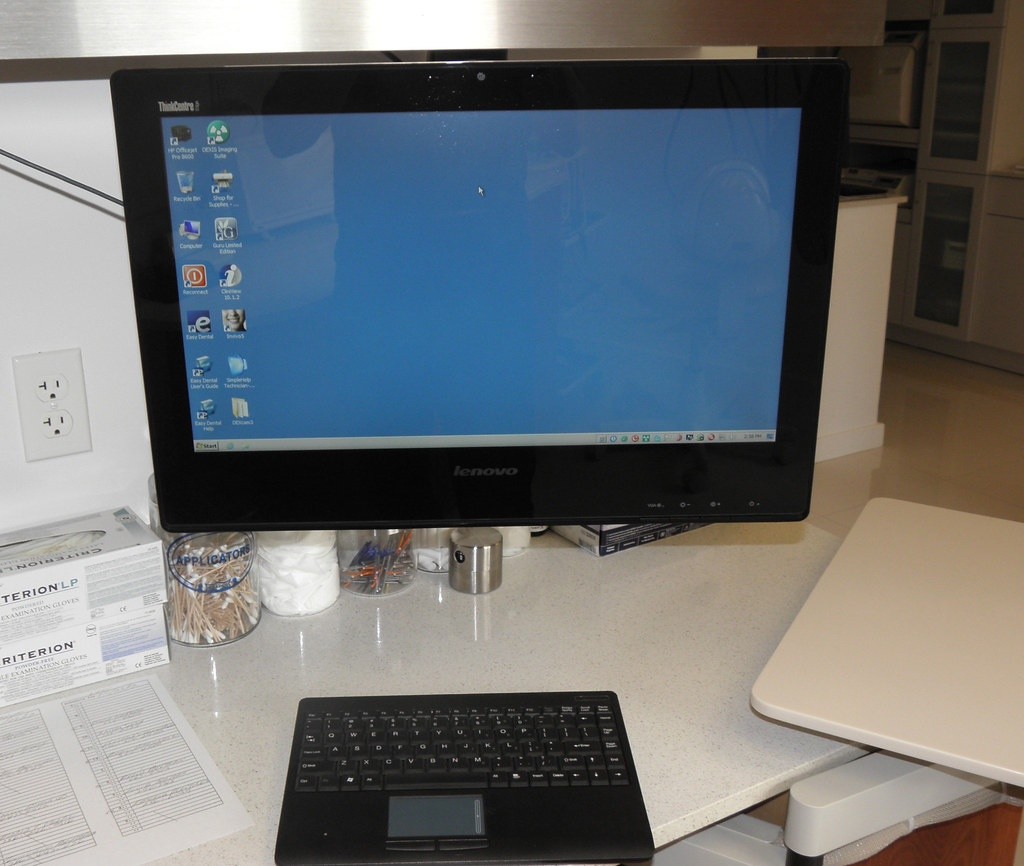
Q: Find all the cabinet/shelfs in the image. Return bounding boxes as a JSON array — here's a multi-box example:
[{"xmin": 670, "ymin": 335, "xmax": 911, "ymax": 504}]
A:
[
  {"xmin": 917, "ymin": 0, "xmax": 1024, "ymax": 176},
  {"xmin": 899, "ymin": 169, "xmax": 1024, "ymax": 375}
]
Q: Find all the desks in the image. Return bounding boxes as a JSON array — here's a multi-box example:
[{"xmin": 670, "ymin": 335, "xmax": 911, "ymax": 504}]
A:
[{"xmin": 750, "ymin": 497, "xmax": 1024, "ymax": 787}]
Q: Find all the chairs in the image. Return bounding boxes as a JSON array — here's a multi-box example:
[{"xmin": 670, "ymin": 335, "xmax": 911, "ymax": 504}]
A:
[{"xmin": 620, "ymin": 750, "xmax": 1023, "ymax": 866}]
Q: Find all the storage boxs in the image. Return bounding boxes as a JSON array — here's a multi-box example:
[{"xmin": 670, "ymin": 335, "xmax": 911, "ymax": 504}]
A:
[{"xmin": 449, "ymin": 527, "xmax": 503, "ymax": 596}]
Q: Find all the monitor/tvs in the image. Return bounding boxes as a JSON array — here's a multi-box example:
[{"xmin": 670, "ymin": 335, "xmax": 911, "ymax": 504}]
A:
[{"xmin": 111, "ymin": 58, "xmax": 852, "ymax": 534}]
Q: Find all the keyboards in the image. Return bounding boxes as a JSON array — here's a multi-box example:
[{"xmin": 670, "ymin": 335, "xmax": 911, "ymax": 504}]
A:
[{"xmin": 274, "ymin": 691, "xmax": 655, "ymax": 866}]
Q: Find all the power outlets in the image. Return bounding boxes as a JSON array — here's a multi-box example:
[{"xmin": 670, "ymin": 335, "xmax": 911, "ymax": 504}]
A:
[{"xmin": 11, "ymin": 347, "xmax": 92, "ymax": 464}]
